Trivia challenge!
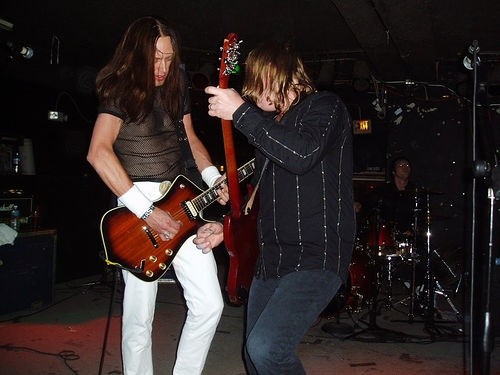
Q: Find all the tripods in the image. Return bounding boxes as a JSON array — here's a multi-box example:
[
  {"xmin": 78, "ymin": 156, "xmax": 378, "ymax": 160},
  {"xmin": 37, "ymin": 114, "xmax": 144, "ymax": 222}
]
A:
[{"xmin": 343, "ymin": 187, "xmax": 465, "ymax": 340}]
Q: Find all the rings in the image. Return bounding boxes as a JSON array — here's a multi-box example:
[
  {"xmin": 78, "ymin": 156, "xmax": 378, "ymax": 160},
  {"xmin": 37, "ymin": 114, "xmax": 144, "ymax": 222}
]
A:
[
  {"xmin": 164, "ymin": 232, "xmax": 170, "ymax": 237},
  {"xmin": 210, "ymin": 104, "xmax": 214, "ymax": 110}
]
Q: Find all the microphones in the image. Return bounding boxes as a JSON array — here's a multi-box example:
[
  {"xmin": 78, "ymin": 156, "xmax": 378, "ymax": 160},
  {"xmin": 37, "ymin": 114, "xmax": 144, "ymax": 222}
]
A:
[{"xmin": 463, "ymin": 40, "xmax": 481, "ymax": 70}]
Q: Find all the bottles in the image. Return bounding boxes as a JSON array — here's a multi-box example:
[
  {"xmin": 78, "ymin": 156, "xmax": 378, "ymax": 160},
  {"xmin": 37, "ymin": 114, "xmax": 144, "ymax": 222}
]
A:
[
  {"xmin": 11, "ymin": 206, "xmax": 19, "ymax": 232},
  {"xmin": 13, "ymin": 153, "xmax": 20, "ymax": 176}
]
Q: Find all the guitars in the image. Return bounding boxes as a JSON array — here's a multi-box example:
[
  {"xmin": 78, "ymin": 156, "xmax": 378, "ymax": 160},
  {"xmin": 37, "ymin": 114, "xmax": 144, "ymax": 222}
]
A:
[
  {"xmin": 99, "ymin": 154, "xmax": 267, "ymax": 282},
  {"xmin": 213, "ymin": 32, "xmax": 265, "ymax": 307}
]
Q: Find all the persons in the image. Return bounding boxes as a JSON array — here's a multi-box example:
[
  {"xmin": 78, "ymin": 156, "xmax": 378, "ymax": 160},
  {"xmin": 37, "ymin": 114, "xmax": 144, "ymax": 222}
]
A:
[
  {"xmin": 358, "ymin": 159, "xmax": 432, "ymax": 314},
  {"xmin": 86, "ymin": 17, "xmax": 232, "ymax": 375},
  {"xmin": 193, "ymin": 45, "xmax": 357, "ymax": 375}
]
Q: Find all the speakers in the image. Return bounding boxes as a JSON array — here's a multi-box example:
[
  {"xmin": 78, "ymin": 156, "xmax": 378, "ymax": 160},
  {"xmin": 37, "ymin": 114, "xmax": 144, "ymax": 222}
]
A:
[{"xmin": 0, "ymin": 228, "xmax": 58, "ymax": 322}]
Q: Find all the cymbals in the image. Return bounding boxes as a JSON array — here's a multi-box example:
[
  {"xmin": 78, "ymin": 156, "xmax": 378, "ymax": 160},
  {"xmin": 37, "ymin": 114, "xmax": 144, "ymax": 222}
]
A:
[{"xmin": 351, "ymin": 170, "xmax": 390, "ymax": 184}]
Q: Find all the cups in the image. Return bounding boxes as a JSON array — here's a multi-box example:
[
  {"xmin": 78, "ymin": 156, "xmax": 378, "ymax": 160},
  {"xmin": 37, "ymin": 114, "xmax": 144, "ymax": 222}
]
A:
[{"xmin": 28, "ymin": 209, "xmax": 40, "ymax": 228}]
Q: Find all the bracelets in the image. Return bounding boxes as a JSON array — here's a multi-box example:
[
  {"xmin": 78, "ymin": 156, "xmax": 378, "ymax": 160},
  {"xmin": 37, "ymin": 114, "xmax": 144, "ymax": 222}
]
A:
[
  {"xmin": 142, "ymin": 205, "xmax": 156, "ymax": 220},
  {"xmin": 118, "ymin": 185, "xmax": 153, "ymax": 219},
  {"xmin": 200, "ymin": 164, "xmax": 223, "ymax": 188}
]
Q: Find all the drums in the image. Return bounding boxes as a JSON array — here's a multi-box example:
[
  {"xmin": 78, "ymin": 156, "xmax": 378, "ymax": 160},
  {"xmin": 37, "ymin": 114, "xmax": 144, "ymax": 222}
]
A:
[
  {"xmin": 315, "ymin": 261, "xmax": 367, "ymax": 319},
  {"xmin": 364, "ymin": 221, "xmax": 401, "ymax": 250}
]
